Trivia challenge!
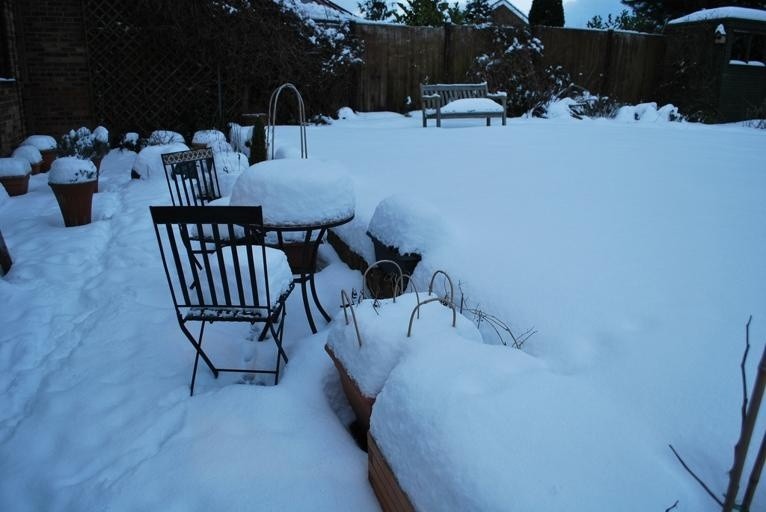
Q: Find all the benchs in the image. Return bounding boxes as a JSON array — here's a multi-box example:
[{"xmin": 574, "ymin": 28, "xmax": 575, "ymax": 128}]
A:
[{"xmin": 419, "ymin": 82, "xmax": 508, "ymax": 128}]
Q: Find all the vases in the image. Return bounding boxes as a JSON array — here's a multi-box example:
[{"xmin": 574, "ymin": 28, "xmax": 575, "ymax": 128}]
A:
[{"xmin": 1, "ymin": 147, "xmax": 56, "ymax": 197}]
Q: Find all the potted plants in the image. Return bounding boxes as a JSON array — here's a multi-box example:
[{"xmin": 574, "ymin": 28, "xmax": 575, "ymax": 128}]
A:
[{"xmin": 47, "ymin": 126, "xmax": 111, "ymax": 227}]
